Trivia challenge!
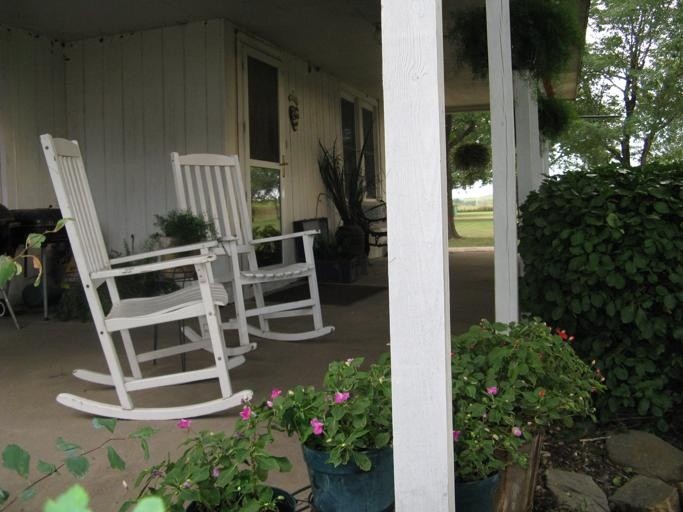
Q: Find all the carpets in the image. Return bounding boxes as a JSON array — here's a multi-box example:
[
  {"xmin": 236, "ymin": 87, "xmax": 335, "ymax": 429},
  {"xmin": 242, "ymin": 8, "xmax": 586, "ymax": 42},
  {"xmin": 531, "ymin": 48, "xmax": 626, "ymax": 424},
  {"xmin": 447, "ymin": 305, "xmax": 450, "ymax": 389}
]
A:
[{"xmin": 252, "ymin": 280, "xmax": 388, "ymax": 306}]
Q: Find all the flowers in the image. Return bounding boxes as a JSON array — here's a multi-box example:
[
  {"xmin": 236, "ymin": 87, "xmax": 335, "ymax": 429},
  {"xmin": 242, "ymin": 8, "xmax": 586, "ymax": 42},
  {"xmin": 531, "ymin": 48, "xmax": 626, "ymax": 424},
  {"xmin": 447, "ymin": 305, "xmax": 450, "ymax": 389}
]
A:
[{"xmin": 152, "ymin": 315, "xmax": 605, "ymax": 511}]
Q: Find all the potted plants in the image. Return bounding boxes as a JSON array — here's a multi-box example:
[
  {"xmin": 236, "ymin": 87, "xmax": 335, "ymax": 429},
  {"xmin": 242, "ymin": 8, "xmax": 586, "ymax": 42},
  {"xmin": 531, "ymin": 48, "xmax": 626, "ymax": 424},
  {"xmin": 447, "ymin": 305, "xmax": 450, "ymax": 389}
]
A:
[{"xmin": 314, "ymin": 138, "xmax": 365, "ymax": 255}]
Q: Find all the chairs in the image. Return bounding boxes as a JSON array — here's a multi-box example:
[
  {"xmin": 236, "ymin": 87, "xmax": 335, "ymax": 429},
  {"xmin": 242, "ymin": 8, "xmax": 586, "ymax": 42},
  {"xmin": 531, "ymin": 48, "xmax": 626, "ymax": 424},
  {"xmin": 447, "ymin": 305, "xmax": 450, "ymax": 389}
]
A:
[
  {"xmin": 39, "ymin": 131, "xmax": 256, "ymax": 420},
  {"xmin": 169, "ymin": 128, "xmax": 335, "ymax": 356}
]
